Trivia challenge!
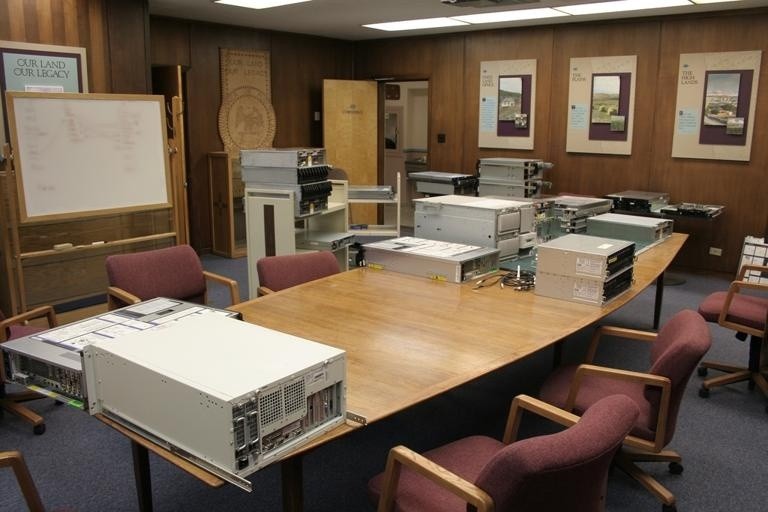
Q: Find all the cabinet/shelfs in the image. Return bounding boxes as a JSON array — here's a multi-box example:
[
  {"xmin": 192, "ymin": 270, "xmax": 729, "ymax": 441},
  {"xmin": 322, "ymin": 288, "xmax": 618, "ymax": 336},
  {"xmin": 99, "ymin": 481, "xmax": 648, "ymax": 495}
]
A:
[
  {"xmin": 349, "ymin": 172, "xmax": 401, "ymax": 254},
  {"xmin": 244, "ymin": 179, "xmax": 349, "ymax": 300}
]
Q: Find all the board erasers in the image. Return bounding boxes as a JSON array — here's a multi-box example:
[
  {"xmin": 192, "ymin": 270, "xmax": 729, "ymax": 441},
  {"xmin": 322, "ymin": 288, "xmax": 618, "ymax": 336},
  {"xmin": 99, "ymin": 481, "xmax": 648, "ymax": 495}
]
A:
[{"xmin": 54, "ymin": 242, "xmax": 72, "ymax": 251}]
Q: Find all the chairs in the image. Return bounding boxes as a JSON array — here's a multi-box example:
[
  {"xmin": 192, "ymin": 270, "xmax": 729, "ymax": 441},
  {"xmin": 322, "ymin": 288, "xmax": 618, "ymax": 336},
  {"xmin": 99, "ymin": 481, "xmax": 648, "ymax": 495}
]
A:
[
  {"xmin": 257, "ymin": 250, "xmax": 339, "ymax": 296},
  {"xmin": 105, "ymin": 245, "xmax": 240, "ymax": 312},
  {"xmin": 366, "ymin": 395, "xmax": 640, "ymax": 512},
  {"xmin": 697, "ymin": 264, "xmax": 768, "ymax": 414},
  {"xmin": 0, "ymin": 305, "xmax": 64, "ymax": 435},
  {"xmin": 0, "ymin": 452, "xmax": 45, "ymax": 512},
  {"xmin": 538, "ymin": 310, "xmax": 710, "ymax": 512}
]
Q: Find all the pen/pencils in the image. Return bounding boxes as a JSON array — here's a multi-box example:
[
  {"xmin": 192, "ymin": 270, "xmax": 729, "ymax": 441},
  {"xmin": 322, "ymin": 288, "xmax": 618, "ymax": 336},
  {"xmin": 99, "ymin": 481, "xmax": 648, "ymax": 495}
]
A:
[{"xmin": 92, "ymin": 241, "xmax": 107, "ymax": 245}]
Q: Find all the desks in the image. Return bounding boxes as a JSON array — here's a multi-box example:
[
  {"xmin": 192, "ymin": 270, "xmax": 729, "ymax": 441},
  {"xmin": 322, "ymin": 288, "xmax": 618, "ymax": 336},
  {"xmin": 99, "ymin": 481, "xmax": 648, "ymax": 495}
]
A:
[
  {"xmin": 613, "ymin": 208, "xmax": 722, "ymax": 285},
  {"xmin": 93, "ymin": 232, "xmax": 689, "ymax": 512}
]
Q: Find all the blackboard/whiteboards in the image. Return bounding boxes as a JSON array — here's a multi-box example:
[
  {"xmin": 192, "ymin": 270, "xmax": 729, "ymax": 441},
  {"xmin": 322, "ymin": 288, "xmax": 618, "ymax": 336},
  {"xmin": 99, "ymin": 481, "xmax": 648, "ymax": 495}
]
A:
[{"xmin": 5, "ymin": 90, "xmax": 174, "ymax": 224}]
